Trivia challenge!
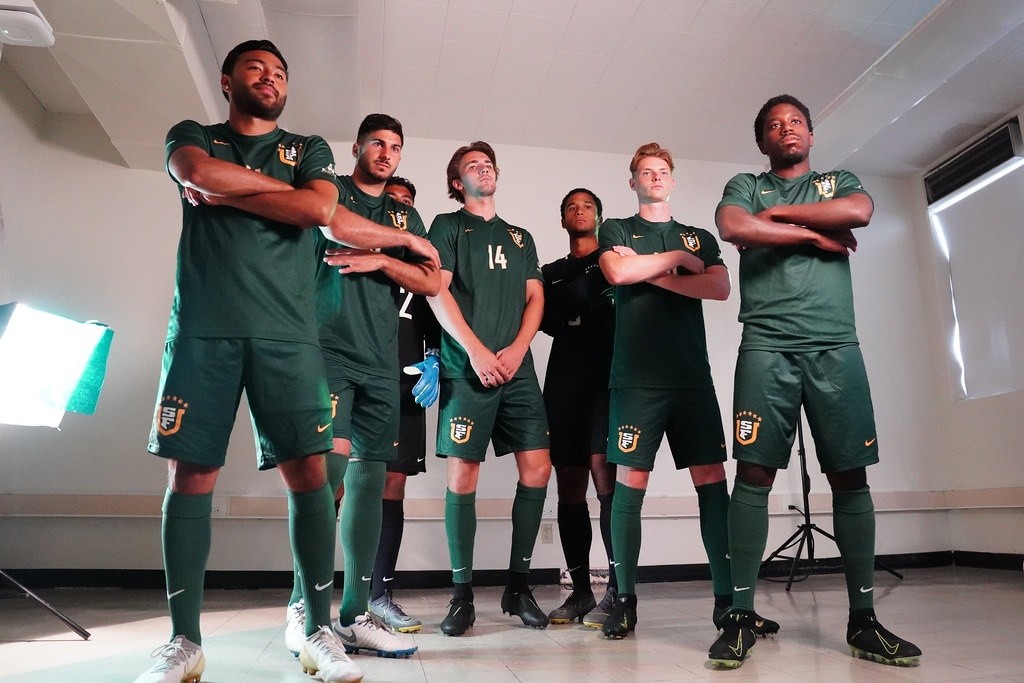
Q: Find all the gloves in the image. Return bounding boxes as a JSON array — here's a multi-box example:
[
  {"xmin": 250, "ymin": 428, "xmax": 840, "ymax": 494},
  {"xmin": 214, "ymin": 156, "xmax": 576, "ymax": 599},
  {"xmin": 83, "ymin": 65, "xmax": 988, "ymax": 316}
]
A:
[{"xmin": 403, "ymin": 349, "xmax": 441, "ymax": 408}]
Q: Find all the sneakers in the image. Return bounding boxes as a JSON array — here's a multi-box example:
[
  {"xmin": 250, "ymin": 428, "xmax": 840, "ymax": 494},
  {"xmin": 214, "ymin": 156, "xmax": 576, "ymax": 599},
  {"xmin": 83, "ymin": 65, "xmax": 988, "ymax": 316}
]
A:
[
  {"xmin": 441, "ymin": 593, "xmax": 475, "ymax": 637},
  {"xmin": 583, "ymin": 587, "xmax": 617, "ymax": 629},
  {"xmin": 286, "ymin": 598, "xmax": 306, "ymax": 657},
  {"xmin": 847, "ymin": 608, "xmax": 922, "ymax": 667},
  {"xmin": 334, "ymin": 612, "xmax": 418, "ymax": 659},
  {"xmin": 713, "ymin": 607, "xmax": 780, "ymax": 636},
  {"xmin": 601, "ymin": 593, "xmax": 637, "ymax": 640},
  {"xmin": 549, "ymin": 590, "xmax": 596, "ymax": 625},
  {"xmin": 501, "ymin": 587, "xmax": 549, "ymax": 629},
  {"xmin": 300, "ymin": 626, "xmax": 364, "ymax": 683},
  {"xmin": 368, "ymin": 591, "xmax": 422, "ymax": 634},
  {"xmin": 133, "ymin": 635, "xmax": 205, "ymax": 683},
  {"xmin": 709, "ymin": 611, "xmax": 757, "ymax": 669}
]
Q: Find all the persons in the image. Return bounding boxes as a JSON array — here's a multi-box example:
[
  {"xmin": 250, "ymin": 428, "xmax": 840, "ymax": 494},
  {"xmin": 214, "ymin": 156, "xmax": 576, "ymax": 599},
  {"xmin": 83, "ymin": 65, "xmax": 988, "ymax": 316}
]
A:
[
  {"xmin": 424, "ymin": 141, "xmax": 551, "ymax": 637},
  {"xmin": 333, "ymin": 170, "xmax": 440, "ymax": 665},
  {"xmin": 533, "ymin": 186, "xmax": 618, "ymax": 630},
  {"xmin": 597, "ymin": 144, "xmax": 782, "ymax": 643},
  {"xmin": 705, "ymin": 95, "xmax": 925, "ymax": 672},
  {"xmin": 282, "ymin": 111, "xmax": 445, "ymax": 658},
  {"xmin": 130, "ymin": 40, "xmax": 366, "ymax": 683}
]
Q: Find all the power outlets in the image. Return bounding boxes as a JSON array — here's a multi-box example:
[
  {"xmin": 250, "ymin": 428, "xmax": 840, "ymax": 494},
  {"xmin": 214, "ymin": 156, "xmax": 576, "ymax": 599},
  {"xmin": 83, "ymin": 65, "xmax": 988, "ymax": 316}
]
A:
[
  {"xmin": 540, "ymin": 524, "xmax": 553, "ymax": 544},
  {"xmin": 212, "ymin": 496, "xmax": 227, "ymax": 517},
  {"xmin": 782, "ymin": 494, "xmax": 804, "ymax": 513}
]
